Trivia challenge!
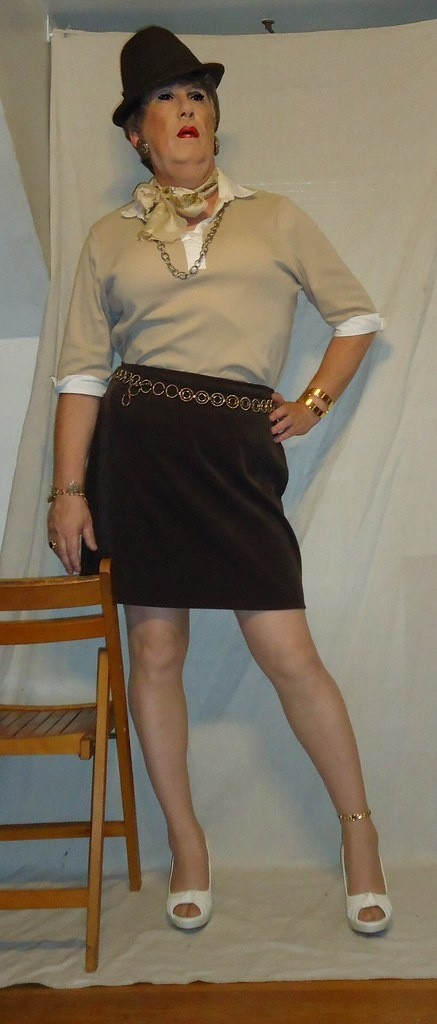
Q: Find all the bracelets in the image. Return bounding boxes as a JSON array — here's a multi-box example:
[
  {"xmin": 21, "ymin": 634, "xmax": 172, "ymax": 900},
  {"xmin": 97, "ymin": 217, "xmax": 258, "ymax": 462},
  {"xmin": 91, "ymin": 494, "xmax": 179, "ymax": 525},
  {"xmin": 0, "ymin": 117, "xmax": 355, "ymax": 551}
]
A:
[
  {"xmin": 47, "ymin": 481, "xmax": 87, "ymax": 503},
  {"xmin": 299, "ymin": 388, "xmax": 334, "ymax": 419}
]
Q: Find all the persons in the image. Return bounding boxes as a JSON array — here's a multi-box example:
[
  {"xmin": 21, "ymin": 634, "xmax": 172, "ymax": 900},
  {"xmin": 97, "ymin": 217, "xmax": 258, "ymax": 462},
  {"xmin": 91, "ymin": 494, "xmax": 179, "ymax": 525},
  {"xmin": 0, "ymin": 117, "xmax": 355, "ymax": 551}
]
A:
[{"xmin": 47, "ymin": 23, "xmax": 394, "ymax": 933}]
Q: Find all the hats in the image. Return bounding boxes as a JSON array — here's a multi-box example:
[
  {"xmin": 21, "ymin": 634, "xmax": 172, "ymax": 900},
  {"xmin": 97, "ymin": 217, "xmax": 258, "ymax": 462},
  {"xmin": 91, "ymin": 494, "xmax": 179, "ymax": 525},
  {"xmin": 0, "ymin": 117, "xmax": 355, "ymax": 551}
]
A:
[{"xmin": 111, "ymin": 26, "xmax": 224, "ymax": 128}]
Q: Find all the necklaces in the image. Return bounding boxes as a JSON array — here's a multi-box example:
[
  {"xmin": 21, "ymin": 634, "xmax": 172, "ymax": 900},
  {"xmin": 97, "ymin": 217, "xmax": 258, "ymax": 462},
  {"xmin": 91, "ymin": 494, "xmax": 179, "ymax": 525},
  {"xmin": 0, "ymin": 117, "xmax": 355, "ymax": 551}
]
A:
[{"xmin": 156, "ymin": 203, "xmax": 228, "ymax": 279}]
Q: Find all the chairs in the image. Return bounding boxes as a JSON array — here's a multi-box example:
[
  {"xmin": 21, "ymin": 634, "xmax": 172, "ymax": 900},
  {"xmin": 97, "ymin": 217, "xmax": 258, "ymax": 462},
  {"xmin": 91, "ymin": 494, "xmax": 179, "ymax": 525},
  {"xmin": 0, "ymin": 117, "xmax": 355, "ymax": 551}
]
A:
[{"xmin": 0, "ymin": 557, "xmax": 141, "ymax": 971}]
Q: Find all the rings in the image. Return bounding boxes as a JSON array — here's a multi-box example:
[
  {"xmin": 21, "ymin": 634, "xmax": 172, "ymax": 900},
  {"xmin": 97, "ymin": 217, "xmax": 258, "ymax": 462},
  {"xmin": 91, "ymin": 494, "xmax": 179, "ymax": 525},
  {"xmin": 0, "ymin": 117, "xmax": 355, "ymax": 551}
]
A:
[{"xmin": 49, "ymin": 541, "xmax": 56, "ymax": 550}]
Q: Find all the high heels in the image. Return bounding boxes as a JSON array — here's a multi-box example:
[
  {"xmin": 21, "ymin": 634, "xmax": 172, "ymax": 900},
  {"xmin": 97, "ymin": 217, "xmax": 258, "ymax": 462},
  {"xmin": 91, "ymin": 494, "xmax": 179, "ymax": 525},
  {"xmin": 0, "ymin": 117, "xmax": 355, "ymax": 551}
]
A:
[
  {"xmin": 341, "ymin": 841, "xmax": 391, "ymax": 933},
  {"xmin": 166, "ymin": 841, "xmax": 212, "ymax": 929}
]
